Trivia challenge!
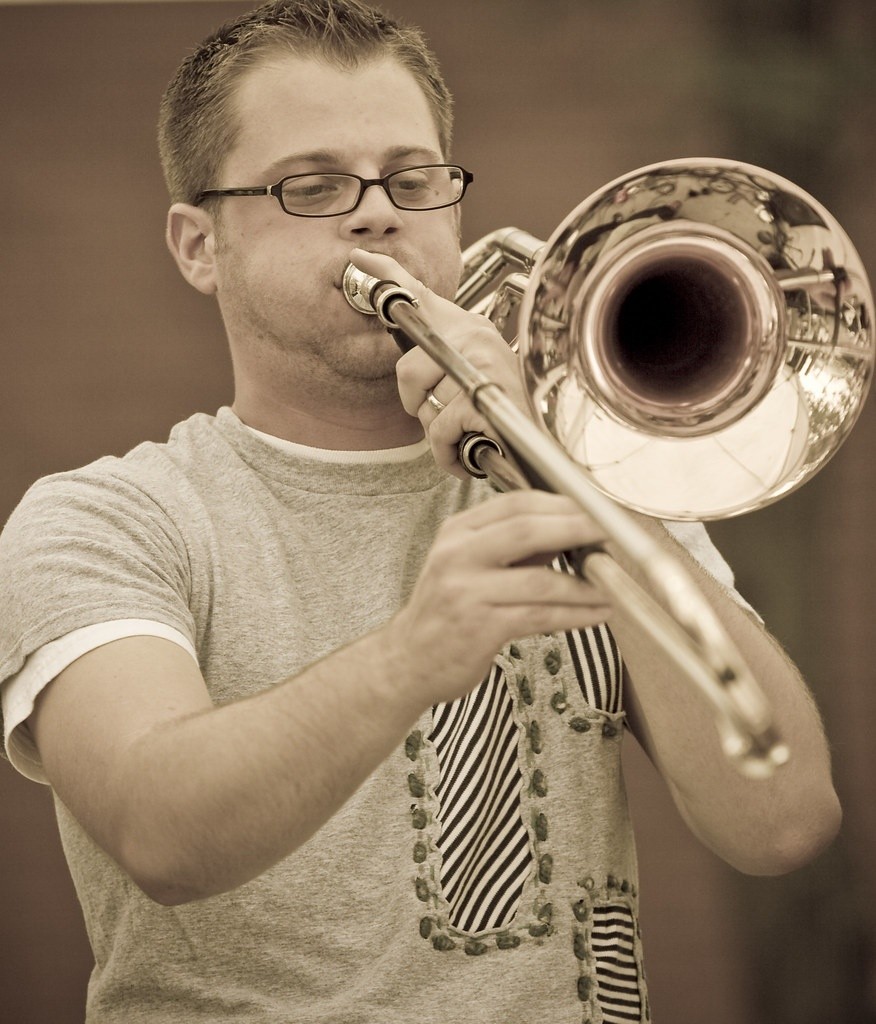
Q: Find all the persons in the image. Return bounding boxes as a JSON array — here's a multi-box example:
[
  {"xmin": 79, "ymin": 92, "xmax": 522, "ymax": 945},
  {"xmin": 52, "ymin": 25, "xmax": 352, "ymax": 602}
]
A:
[{"xmin": 1, "ymin": 1, "xmax": 842, "ymax": 1024}]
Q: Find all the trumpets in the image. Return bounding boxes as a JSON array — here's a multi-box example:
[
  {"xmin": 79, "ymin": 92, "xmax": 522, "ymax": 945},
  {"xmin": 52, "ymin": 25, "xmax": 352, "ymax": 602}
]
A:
[{"xmin": 340, "ymin": 153, "xmax": 876, "ymax": 788}]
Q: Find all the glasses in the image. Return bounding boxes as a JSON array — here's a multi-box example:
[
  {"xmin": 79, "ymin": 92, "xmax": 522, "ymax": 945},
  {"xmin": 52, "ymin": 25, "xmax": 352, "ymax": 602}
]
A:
[{"xmin": 187, "ymin": 160, "xmax": 477, "ymax": 222}]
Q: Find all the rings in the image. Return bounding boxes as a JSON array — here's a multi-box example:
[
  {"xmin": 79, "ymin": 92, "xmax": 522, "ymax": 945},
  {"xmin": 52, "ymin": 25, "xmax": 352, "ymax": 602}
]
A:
[{"xmin": 424, "ymin": 390, "xmax": 446, "ymax": 413}]
